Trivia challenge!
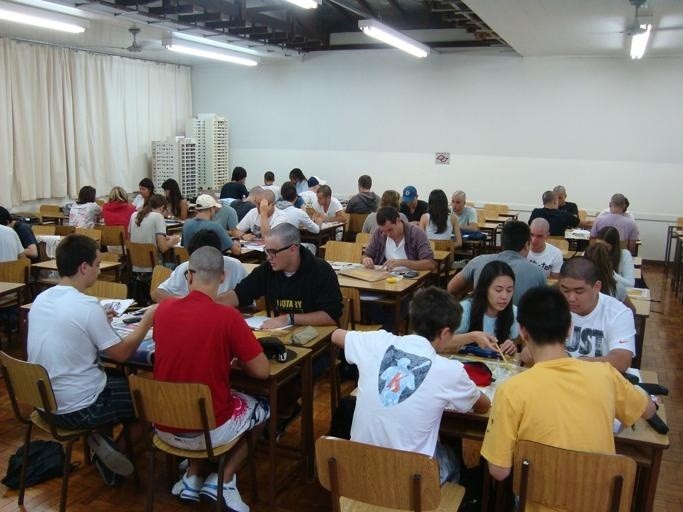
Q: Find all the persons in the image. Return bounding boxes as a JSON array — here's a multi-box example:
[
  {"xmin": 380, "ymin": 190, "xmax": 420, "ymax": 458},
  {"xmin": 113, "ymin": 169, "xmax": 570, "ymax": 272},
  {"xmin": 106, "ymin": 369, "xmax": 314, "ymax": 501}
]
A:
[
  {"xmin": 479, "ymin": 286, "xmax": 661, "ymax": 512},
  {"xmin": 331, "ymin": 285, "xmax": 490, "ymax": 488},
  {"xmin": 520, "ymin": 255, "xmax": 636, "ymax": 375},
  {"xmin": 215, "ymin": 222, "xmax": 344, "ymax": 330},
  {"xmin": 150, "ymin": 226, "xmax": 257, "ymax": 308},
  {"xmin": 448, "ymin": 220, "xmax": 547, "ymax": 306},
  {"xmin": 583, "ymin": 192, "xmax": 638, "ymax": 311},
  {"xmin": 69, "ymin": 177, "xmax": 190, "ymax": 283},
  {"xmin": 183, "ymin": 167, "xmax": 345, "ymax": 258},
  {"xmin": 153, "ymin": 245, "xmax": 270, "ymax": 510},
  {"xmin": 527, "ymin": 185, "xmax": 587, "ymax": 235},
  {"xmin": 1, "ymin": 207, "xmax": 40, "ymax": 332},
  {"xmin": 527, "ymin": 217, "xmax": 563, "ymax": 278},
  {"xmin": 346, "ymin": 175, "xmax": 477, "ymax": 269},
  {"xmin": 442, "ymin": 260, "xmax": 520, "ymax": 356},
  {"xmin": 26, "ymin": 234, "xmax": 159, "ymax": 488}
]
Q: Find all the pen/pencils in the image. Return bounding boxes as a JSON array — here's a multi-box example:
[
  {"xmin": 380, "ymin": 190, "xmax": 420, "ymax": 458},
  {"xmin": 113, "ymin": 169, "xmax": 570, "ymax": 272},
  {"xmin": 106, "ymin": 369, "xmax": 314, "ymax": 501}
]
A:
[{"xmin": 496, "ymin": 342, "xmax": 508, "ymax": 362}]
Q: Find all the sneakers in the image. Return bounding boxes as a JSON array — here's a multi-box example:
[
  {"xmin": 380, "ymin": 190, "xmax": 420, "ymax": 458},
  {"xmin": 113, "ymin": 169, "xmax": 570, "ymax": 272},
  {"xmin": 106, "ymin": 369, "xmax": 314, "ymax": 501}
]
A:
[
  {"xmin": 200, "ymin": 473, "xmax": 251, "ymax": 512},
  {"xmin": 260, "ymin": 404, "xmax": 302, "ymax": 445},
  {"xmin": 86, "ymin": 432, "xmax": 135, "ymax": 487},
  {"xmin": 171, "ymin": 468, "xmax": 203, "ymax": 502},
  {"xmin": 7, "ymin": 318, "xmax": 26, "ymax": 333}
]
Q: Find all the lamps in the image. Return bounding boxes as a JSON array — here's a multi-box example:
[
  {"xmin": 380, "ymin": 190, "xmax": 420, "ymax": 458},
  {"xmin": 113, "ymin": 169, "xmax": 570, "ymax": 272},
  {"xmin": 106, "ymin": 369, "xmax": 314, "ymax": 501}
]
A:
[
  {"xmin": 358, "ymin": 19, "xmax": 431, "ymax": 58},
  {"xmin": 162, "ymin": 39, "xmax": 260, "ymax": 67},
  {"xmin": 0, "ymin": 2, "xmax": 89, "ymax": 34},
  {"xmin": 285, "ymin": 0, "xmax": 322, "ymax": 9},
  {"xmin": 626, "ymin": 15, "xmax": 652, "ymax": 62}
]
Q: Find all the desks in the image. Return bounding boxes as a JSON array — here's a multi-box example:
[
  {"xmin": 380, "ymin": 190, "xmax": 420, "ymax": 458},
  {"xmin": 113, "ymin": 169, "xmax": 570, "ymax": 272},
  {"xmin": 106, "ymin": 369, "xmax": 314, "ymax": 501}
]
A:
[
  {"xmin": 247, "ymin": 319, "xmax": 340, "ymax": 449},
  {"xmin": 92, "ymin": 323, "xmax": 315, "ymax": 498},
  {"xmin": 436, "ymin": 351, "xmax": 662, "ymax": 401},
  {"xmin": 351, "ymin": 372, "xmax": 669, "ymax": 508},
  {"xmin": 0, "ymin": 190, "xmax": 649, "ymax": 365}
]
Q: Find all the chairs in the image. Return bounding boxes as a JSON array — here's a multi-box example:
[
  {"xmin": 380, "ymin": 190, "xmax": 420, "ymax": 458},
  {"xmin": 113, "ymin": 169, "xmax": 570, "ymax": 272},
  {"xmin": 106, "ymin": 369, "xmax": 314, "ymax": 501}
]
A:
[
  {"xmin": 127, "ymin": 375, "xmax": 254, "ymax": 511},
  {"xmin": 315, "ymin": 437, "xmax": 464, "ymax": 512},
  {"xmin": 513, "ymin": 439, "xmax": 636, "ymax": 512},
  {"xmin": 1, "ymin": 351, "xmax": 139, "ymax": 510}
]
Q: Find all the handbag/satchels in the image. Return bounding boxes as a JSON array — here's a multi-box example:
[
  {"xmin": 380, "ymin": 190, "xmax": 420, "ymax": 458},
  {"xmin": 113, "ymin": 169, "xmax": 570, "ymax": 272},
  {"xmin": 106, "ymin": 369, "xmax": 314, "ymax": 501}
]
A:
[{"xmin": 1, "ymin": 440, "xmax": 74, "ymax": 489}]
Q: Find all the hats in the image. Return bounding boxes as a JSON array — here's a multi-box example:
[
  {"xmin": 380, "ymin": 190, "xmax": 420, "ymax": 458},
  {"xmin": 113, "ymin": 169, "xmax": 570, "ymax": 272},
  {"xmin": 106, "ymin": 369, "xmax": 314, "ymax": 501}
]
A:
[
  {"xmin": 195, "ymin": 194, "xmax": 222, "ymax": 209},
  {"xmin": 257, "ymin": 337, "xmax": 298, "ymax": 362},
  {"xmin": 308, "ymin": 176, "xmax": 326, "ymax": 187},
  {"xmin": 402, "ymin": 186, "xmax": 416, "ymax": 201}
]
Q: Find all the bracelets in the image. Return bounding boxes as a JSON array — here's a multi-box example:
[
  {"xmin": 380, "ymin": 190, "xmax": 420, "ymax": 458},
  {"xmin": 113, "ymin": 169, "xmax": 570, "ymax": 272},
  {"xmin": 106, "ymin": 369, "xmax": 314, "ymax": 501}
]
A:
[
  {"xmin": 654, "ymin": 402, "xmax": 660, "ymax": 411},
  {"xmin": 287, "ymin": 312, "xmax": 295, "ymax": 325}
]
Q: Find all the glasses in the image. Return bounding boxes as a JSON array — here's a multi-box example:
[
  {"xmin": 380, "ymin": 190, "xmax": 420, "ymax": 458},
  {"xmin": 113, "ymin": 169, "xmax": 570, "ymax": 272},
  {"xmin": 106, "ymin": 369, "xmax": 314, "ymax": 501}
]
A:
[
  {"xmin": 263, "ymin": 244, "xmax": 299, "ymax": 258},
  {"xmin": 183, "ymin": 269, "xmax": 195, "ymax": 279}
]
